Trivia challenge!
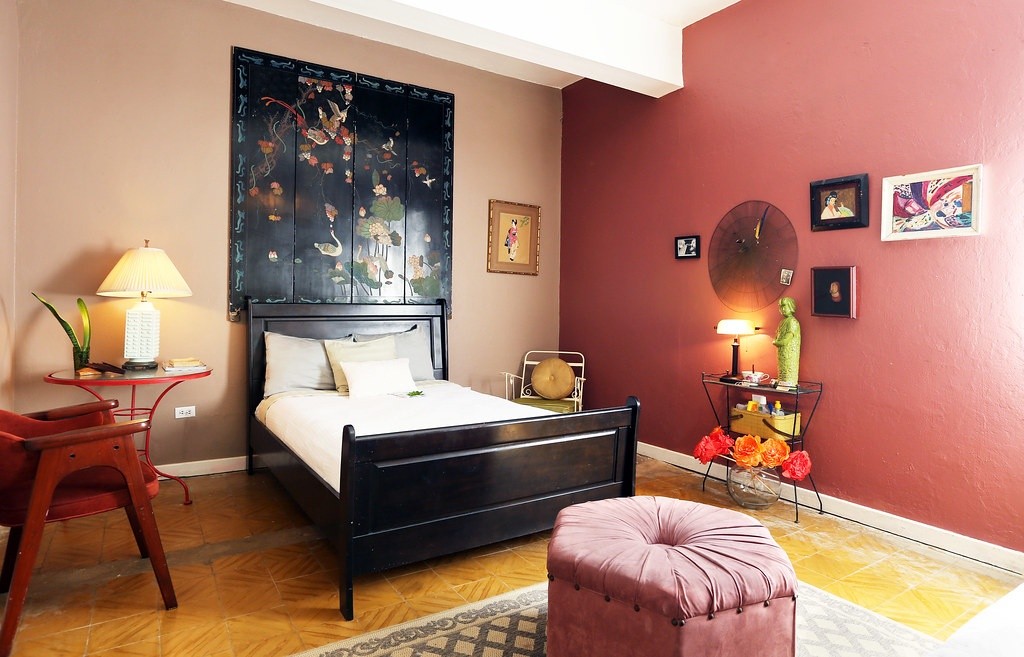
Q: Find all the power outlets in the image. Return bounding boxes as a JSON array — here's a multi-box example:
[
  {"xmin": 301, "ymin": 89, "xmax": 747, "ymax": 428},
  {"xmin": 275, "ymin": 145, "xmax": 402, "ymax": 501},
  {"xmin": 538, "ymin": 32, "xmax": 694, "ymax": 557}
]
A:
[{"xmin": 175, "ymin": 406, "xmax": 196, "ymax": 418}]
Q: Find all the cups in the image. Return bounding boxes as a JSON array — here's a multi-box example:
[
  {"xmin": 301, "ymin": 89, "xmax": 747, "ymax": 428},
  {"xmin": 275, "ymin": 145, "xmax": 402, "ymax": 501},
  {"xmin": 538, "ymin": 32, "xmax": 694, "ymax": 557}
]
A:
[{"xmin": 741, "ymin": 371, "xmax": 770, "ymax": 386}]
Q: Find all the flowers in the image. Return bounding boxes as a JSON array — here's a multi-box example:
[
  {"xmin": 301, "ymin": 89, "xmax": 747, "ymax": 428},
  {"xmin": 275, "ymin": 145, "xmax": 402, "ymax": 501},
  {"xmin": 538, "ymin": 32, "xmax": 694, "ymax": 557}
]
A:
[{"xmin": 694, "ymin": 426, "xmax": 812, "ymax": 496}]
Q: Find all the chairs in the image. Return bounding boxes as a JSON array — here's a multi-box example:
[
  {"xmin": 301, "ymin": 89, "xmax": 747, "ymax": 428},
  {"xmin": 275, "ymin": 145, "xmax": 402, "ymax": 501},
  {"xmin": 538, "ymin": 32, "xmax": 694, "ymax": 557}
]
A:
[
  {"xmin": 499, "ymin": 350, "xmax": 586, "ymax": 414},
  {"xmin": 0, "ymin": 398, "xmax": 178, "ymax": 657}
]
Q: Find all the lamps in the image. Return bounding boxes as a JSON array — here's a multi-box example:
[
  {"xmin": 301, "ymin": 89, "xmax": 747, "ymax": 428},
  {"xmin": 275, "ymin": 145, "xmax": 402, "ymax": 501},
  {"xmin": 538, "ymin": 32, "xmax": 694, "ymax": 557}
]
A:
[
  {"xmin": 95, "ymin": 239, "xmax": 193, "ymax": 370},
  {"xmin": 713, "ymin": 319, "xmax": 761, "ymax": 383}
]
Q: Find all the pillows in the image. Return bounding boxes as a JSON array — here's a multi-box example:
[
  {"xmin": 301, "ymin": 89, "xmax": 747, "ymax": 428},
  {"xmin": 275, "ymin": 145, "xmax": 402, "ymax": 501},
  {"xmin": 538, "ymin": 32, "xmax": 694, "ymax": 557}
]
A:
[
  {"xmin": 530, "ymin": 357, "xmax": 574, "ymax": 400},
  {"xmin": 260, "ymin": 326, "xmax": 434, "ymax": 399}
]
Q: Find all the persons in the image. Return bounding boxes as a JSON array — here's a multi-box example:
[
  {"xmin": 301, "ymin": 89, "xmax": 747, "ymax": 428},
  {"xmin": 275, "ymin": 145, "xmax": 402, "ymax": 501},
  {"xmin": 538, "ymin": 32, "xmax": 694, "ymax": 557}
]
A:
[{"xmin": 772, "ymin": 297, "xmax": 801, "ymax": 384}]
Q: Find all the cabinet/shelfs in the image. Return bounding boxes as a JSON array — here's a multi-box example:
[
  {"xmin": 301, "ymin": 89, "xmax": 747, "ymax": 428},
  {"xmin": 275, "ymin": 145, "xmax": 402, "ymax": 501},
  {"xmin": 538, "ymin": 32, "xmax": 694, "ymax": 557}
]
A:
[{"xmin": 701, "ymin": 371, "xmax": 823, "ymax": 523}]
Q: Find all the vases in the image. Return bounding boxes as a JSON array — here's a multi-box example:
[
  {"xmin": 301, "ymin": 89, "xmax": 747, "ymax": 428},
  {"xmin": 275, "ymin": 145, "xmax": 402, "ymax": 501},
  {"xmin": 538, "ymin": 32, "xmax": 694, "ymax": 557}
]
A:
[{"xmin": 727, "ymin": 462, "xmax": 781, "ymax": 509}]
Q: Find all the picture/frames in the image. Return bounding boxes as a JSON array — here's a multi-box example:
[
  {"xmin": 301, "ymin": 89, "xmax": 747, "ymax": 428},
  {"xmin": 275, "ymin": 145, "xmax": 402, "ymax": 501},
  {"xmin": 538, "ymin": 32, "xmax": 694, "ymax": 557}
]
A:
[
  {"xmin": 882, "ymin": 164, "xmax": 984, "ymax": 242},
  {"xmin": 810, "ymin": 174, "xmax": 869, "ymax": 232},
  {"xmin": 810, "ymin": 265, "xmax": 857, "ymax": 319},
  {"xmin": 487, "ymin": 199, "xmax": 542, "ymax": 276},
  {"xmin": 675, "ymin": 235, "xmax": 701, "ymax": 260}
]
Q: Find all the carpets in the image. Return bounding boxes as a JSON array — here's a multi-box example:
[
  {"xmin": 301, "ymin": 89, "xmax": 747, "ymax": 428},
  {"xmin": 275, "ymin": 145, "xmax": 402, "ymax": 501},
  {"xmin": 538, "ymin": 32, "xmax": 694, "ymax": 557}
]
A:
[{"xmin": 292, "ymin": 575, "xmax": 944, "ymax": 657}]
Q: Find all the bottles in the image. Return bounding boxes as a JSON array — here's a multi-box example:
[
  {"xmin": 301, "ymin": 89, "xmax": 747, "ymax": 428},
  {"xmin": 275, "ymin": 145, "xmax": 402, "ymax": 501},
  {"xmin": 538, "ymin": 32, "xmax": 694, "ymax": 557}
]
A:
[{"xmin": 774, "ymin": 401, "xmax": 784, "ymax": 417}]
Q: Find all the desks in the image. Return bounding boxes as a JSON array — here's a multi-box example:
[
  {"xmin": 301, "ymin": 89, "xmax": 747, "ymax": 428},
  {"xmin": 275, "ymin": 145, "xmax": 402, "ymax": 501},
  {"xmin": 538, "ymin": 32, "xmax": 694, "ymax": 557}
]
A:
[{"xmin": 44, "ymin": 362, "xmax": 213, "ymax": 505}]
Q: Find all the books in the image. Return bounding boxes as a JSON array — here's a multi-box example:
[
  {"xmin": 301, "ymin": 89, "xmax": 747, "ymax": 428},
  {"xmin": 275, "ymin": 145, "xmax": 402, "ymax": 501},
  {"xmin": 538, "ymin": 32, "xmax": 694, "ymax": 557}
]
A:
[{"xmin": 162, "ymin": 361, "xmax": 207, "ymax": 372}]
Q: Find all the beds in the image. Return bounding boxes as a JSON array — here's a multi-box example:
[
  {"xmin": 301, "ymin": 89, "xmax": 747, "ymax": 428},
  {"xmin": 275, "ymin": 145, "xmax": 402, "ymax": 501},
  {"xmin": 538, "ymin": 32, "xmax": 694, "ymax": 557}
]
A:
[{"xmin": 246, "ymin": 296, "xmax": 640, "ymax": 621}]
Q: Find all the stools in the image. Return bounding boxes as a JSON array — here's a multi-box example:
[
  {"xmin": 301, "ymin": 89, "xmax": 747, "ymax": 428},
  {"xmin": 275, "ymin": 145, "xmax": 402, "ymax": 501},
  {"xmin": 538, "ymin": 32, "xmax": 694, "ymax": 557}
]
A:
[{"xmin": 546, "ymin": 496, "xmax": 799, "ymax": 657}]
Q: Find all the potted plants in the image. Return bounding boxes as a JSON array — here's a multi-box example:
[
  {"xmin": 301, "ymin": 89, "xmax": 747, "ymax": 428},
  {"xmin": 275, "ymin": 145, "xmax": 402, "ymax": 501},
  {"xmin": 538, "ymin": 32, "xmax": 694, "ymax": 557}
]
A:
[{"xmin": 31, "ymin": 292, "xmax": 91, "ymax": 372}]
